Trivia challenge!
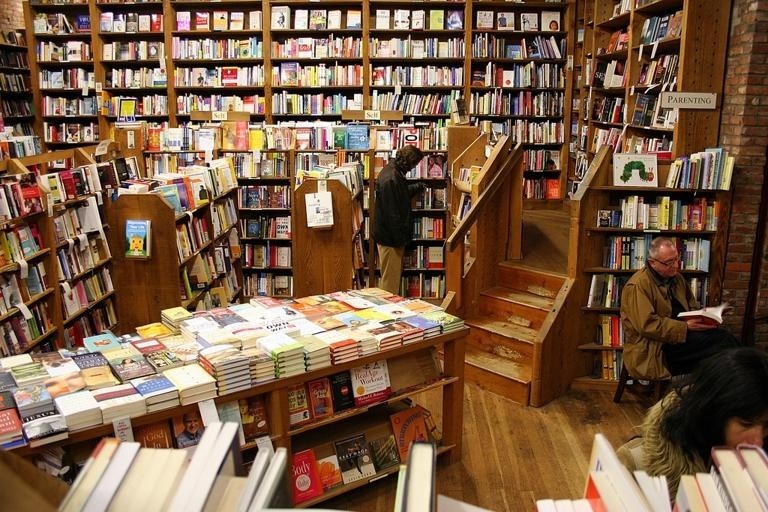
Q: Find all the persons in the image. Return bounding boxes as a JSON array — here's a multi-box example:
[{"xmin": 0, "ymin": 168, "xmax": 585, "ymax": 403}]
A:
[
  {"xmin": 621, "ymin": 236, "xmax": 743, "ymax": 389},
  {"xmin": 616, "ymin": 345, "xmax": 768, "ymax": 511}
]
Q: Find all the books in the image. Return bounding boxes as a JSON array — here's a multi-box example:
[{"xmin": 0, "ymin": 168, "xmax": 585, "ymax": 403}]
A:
[
  {"xmin": 1, "ymin": 1, "xmax": 482, "ymax": 354},
  {"xmin": 568, "ymin": 428, "xmax": 767, "ymax": 512},
  {"xmin": 589, "ymin": 148, "xmax": 734, "ymax": 381},
  {"xmin": 469, "ymin": 10, "xmax": 565, "ymax": 199},
  {"xmin": 535, "ymin": 498, "xmax": 594, "ymax": 512},
  {"xmin": 569, "ymin": 0, "xmax": 683, "ymax": 195},
  {"xmin": 0, "ymin": 284, "xmax": 464, "ymax": 512}
]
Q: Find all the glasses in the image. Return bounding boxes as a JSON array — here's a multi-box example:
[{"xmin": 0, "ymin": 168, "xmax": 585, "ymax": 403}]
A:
[{"xmin": 650, "ymin": 254, "xmax": 683, "ymax": 266}]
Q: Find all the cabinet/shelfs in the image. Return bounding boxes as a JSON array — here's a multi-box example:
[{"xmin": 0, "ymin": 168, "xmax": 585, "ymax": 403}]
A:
[
  {"xmin": 2, "ymin": 1, "xmax": 732, "ymax": 357},
  {"xmin": 3, "ymin": 287, "xmax": 471, "ymax": 507},
  {"xmin": 571, "ymin": 162, "xmax": 732, "ymax": 389}
]
